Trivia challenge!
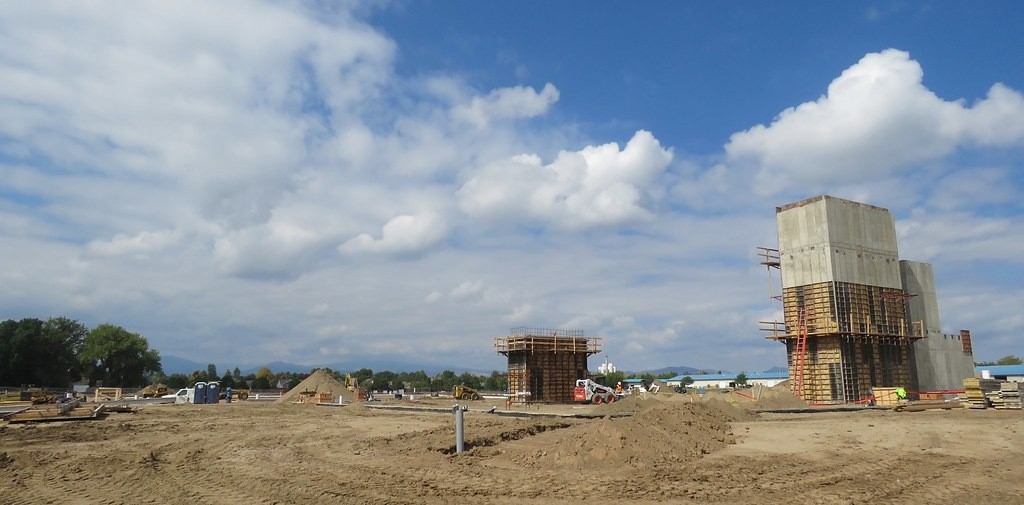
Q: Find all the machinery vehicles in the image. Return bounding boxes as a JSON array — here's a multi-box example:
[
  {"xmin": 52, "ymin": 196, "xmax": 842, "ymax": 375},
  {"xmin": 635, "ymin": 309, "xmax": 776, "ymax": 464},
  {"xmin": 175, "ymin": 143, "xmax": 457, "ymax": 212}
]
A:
[
  {"xmin": 345, "ymin": 372, "xmax": 358, "ymax": 393},
  {"xmin": 575, "ymin": 378, "xmax": 616, "ymax": 405},
  {"xmin": 453, "ymin": 385, "xmax": 478, "ymax": 400}
]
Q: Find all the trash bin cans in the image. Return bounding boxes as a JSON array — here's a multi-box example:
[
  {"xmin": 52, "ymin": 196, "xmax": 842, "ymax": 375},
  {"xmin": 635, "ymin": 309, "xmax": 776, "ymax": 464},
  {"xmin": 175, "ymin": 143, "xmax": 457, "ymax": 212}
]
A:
[{"xmin": 395, "ymin": 394, "xmax": 402, "ymax": 400}]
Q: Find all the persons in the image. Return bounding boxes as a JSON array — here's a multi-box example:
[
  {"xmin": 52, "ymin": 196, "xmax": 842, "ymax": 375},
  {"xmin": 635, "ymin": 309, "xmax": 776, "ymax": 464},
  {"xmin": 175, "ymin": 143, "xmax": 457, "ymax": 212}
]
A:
[
  {"xmin": 615, "ymin": 381, "xmax": 623, "ymax": 394},
  {"xmin": 866, "ymin": 386, "xmax": 875, "ymax": 407}
]
